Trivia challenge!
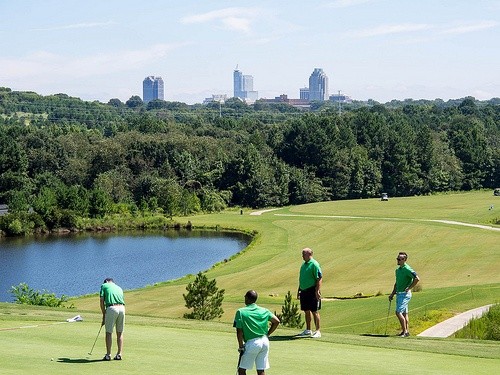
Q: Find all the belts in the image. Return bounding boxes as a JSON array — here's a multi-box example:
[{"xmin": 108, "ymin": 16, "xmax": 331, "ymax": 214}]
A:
[
  {"xmin": 109, "ymin": 303, "xmax": 124, "ymax": 306},
  {"xmin": 396, "ymin": 292, "xmax": 406, "ymax": 294}
]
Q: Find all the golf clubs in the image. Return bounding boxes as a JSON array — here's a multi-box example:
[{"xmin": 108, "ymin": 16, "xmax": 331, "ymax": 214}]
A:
[
  {"xmin": 384, "ymin": 300, "xmax": 392, "ymax": 337},
  {"xmin": 235, "ymin": 350, "xmax": 243, "ymax": 375},
  {"xmin": 88, "ymin": 324, "xmax": 104, "ymax": 355}
]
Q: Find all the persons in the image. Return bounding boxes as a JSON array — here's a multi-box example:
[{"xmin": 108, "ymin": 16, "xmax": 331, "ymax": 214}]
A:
[
  {"xmin": 297, "ymin": 248, "xmax": 323, "ymax": 338},
  {"xmin": 389, "ymin": 252, "xmax": 420, "ymax": 336},
  {"xmin": 99, "ymin": 278, "xmax": 125, "ymax": 360},
  {"xmin": 233, "ymin": 290, "xmax": 280, "ymax": 375}
]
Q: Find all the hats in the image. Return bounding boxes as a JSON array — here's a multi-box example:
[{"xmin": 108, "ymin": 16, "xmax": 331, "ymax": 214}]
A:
[{"xmin": 103, "ymin": 278, "xmax": 112, "ymax": 284}]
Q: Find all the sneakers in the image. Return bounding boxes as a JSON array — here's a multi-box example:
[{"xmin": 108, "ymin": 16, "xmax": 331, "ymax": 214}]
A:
[
  {"xmin": 298, "ymin": 330, "xmax": 312, "ymax": 336},
  {"xmin": 308, "ymin": 330, "xmax": 321, "ymax": 338}
]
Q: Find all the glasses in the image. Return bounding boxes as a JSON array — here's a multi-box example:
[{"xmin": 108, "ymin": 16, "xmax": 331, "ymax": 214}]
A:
[{"xmin": 397, "ymin": 258, "xmax": 401, "ymax": 261}]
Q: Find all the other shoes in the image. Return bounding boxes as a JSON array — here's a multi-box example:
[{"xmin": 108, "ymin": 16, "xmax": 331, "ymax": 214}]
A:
[
  {"xmin": 104, "ymin": 354, "xmax": 111, "ymax": 360},
  {"xmin": 398, "ymin": 330, "xmax": 409, "ymax": 337},
  {"xmin": 114, "ymin": 354, "xmax": 122, "ymax": 360}
]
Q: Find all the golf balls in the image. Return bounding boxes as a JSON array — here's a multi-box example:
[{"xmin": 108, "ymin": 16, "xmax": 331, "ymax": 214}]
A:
[{"xmin": 51, "ymin": 358, "xmax": 53, "ymax": 361}]
